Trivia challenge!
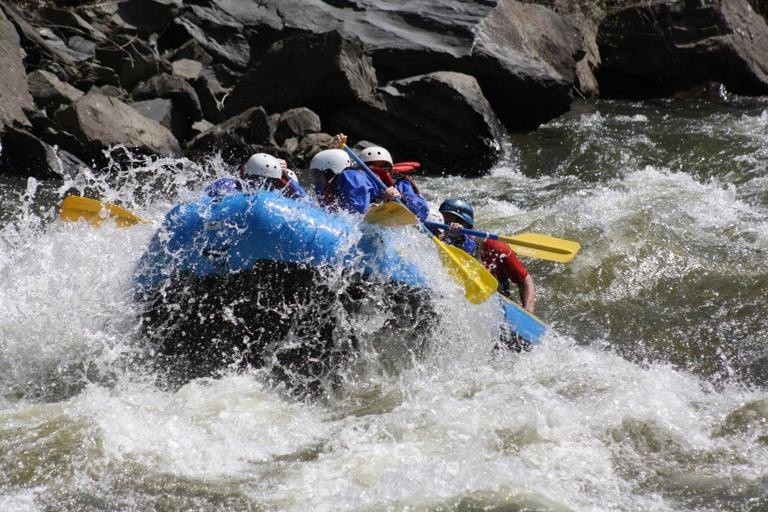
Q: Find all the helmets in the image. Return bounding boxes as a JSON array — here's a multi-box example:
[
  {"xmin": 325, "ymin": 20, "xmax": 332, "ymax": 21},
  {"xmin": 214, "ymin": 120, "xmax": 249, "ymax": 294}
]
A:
[
  {"xmin": 207, "ymin": 178, "xmax": 244, "ymax": 197},
  {"xmin": 359, "ymin": 146, "xmax": 394, "ymax": 171},
  {"xmin": 438, "ymin": 197, "xmax": 474, "ymax": 228},
  {"xmin": 425, "ymin": 210, "xmax": 446, "ymax": 234},
  {"xmin": 244, "ymin": 152, "xmax": 282, "ymax": 180},
  {"xmin": 309, "ymin": 148, "xmax": 351, "ymax": 176},
  {"xmin": 285, "ymin": 169, "xmax": 299, "ymax": 182}
]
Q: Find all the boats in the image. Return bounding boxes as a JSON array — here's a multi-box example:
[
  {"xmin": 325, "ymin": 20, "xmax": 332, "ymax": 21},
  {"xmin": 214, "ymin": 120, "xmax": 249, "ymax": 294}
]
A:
[{"xmin": 131, "ymin": 191, "xmax": 563, "ymax": 374}]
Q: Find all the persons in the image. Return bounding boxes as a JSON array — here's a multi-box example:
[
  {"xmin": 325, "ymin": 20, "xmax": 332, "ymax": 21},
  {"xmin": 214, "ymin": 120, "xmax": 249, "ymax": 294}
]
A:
[{"xmin": 204, "ymin": 129, "xmax": 537, "ymax": 318}]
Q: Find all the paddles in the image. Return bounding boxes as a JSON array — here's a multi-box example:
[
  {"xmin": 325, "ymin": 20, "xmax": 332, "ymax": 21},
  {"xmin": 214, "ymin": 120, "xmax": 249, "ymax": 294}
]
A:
[
  {"xmin": 422, "ymin": 221, "xmax": 580, "ymax": 262},
  {"xmin": 60, "ymin": 194, "xmax": 153, "ymax": 229},
  {"xmin": 333, "ymin": 137, "xmax": 499, "ymax": 305},
  {"xmin": 363, "ymin": 201, "xmax": 418, "ymax": 227}
]
